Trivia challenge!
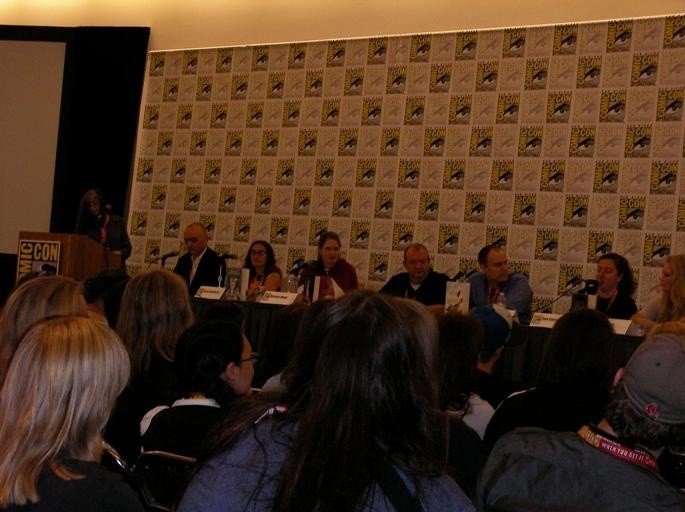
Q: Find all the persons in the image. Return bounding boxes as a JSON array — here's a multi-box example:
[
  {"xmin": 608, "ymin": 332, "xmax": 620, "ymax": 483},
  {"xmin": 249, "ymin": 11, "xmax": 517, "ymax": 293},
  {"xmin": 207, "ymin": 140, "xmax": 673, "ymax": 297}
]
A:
[
  {"xmin": 1, "ymin": 276, "xmax": 91, "ymax": 380},
  {"xmin": 0, "ymin": 318, "xmax": 153, "ymax": 512},
  {"xmin": 127, "ymin": 318, "xmax": 260, "ymax": 459},
  {"xmin": 482, "ymin": 308, "xmax": 624, "ymax": 458},
  {"xmin": 571, "ymin": 251, "xmax": 638, "ymax": 319},
  {"xmin": 464, "ymin": 244, "xmax": 533, "ymax": 324},
  {"xmin": 378, "ymin": 243, "xmax": 452, "ymax": 306},
  {"xmin": 237, "ymin": 240, "xmax": 282, "ymax": 298},
  {"xmin": 473, "ymin": 330, "xmax": 685, "ymax": 511},
  {"xmin": 459, "ymin": 307, "xmax": 512, "ymax": 403},
  {"xmin": 181, "ymin": 293, "xmax": 472, "ymax": 511},
  {"xmin": 174, "ymin": 223, "xmax": 227, "ymax": 295},
  {"xmin": 297, "ymin": 232, "xmax": 358, "ymax": 300},
  {"xmin": 74, "ymin": 187, "xmax": 133, "ymax": 263},
  {"xmin": 107, "ymin": 269, "xmax": 195, "ymax": 452},
  {"xmin": 631, "ymin": 254, "xmax": 684, "ymax": 335}
]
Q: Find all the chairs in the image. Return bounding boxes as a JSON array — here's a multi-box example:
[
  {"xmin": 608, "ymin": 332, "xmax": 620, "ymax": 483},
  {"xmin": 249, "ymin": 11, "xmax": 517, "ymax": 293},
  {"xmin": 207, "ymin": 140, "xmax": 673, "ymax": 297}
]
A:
[{"xmin": 132, "ymin": 450, "xmax": 198, "ymax": 512}]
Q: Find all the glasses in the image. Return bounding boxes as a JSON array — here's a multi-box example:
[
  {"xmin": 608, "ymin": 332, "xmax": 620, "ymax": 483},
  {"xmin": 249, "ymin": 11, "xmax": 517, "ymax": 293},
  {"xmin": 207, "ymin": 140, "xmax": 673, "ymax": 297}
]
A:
[
  {"xmin": 235, "ymin": 352, "xmax": 259, "ymax": 364},
  {"xmin": 251, "ymin": 251, "xmax": 266, "ymax": 255}
]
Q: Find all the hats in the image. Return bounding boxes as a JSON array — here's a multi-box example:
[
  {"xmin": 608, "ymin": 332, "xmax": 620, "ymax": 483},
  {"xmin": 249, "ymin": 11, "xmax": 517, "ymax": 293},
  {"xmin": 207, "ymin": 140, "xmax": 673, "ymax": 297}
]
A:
[{"xmin": 622, "ymin": 334, "xmax": 685, "ymax": 424}]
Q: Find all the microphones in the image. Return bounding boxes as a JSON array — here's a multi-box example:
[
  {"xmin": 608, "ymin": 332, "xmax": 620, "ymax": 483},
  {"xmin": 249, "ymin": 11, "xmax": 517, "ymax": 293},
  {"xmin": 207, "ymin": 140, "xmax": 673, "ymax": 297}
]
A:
[
  {"xmin": 98, "ymin": 204, "xmax": 112, "ymax": 219},
  {"xmin": 292, "ymin": 261, "xmax": 312, "ymax": 274},
  {"xmin": 162, "ymin": 250, "xmax": 181, "ymax": 260},
  {"xmin": 220, "ymin": 254, "xmax": 236, "ymax": 260}
]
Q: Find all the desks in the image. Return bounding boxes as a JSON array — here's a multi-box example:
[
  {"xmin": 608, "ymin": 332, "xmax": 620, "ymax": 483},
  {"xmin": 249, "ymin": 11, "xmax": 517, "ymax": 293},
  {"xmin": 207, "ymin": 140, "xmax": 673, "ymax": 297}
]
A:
[
  {"xmin": 191, "ymin": 296, "xmax": 647, "ymax": 394},
  {"xmin": 16, "ymin": 231, "xmax": 122, "ymax": 288}
]
